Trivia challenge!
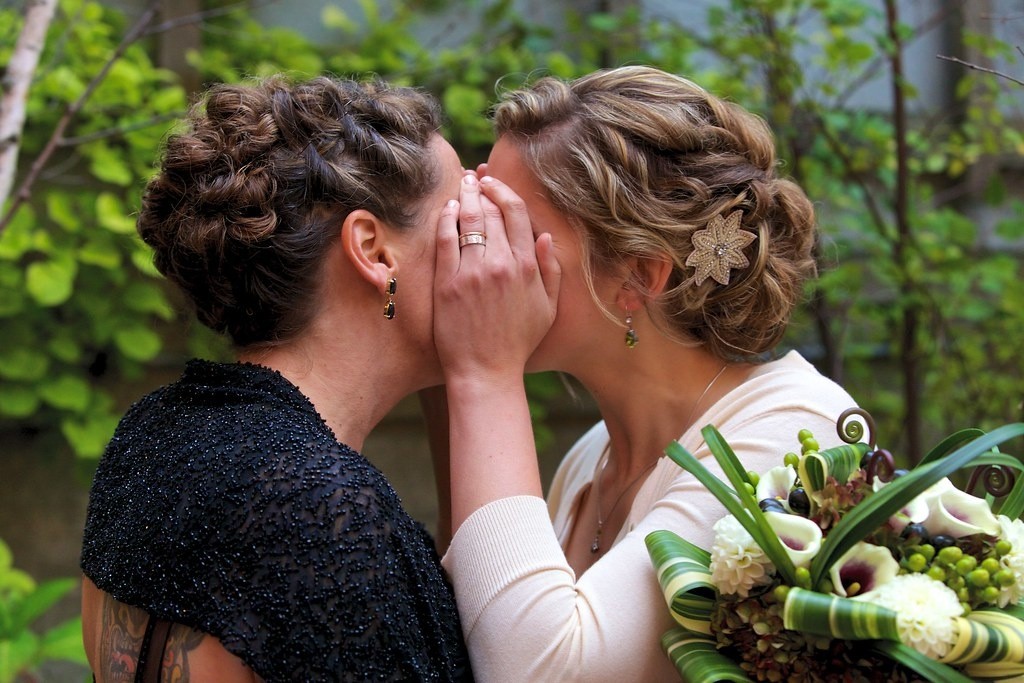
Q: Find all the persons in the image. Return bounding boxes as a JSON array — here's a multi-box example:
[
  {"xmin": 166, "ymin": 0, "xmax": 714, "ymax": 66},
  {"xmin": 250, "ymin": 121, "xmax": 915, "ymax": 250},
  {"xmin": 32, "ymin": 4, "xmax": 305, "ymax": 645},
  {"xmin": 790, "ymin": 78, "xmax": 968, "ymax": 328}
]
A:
[
  {"xmin": 432, "ymin": 65, "xmax": 879, "ymax": 683},
  {"xmin": 79, "ymin": 71, "xmax": 477, "ymax": 683}
]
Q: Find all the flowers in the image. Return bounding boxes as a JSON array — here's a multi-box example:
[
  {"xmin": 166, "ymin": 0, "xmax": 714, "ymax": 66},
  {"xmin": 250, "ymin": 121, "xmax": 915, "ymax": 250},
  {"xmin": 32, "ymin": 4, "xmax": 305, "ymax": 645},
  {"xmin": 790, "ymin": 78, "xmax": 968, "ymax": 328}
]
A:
[{"xmin": 642, "ymin": 405, "xmax": 1024, "ymax": 683}]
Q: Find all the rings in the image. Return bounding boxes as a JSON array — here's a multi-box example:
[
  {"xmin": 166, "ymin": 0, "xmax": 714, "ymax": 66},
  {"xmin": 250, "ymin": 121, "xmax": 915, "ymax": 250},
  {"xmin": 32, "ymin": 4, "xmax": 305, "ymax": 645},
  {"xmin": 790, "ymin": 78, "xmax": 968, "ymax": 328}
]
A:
[{"xmin": 459, "ymin": 232, "xmax": 486, "ymax": 247}]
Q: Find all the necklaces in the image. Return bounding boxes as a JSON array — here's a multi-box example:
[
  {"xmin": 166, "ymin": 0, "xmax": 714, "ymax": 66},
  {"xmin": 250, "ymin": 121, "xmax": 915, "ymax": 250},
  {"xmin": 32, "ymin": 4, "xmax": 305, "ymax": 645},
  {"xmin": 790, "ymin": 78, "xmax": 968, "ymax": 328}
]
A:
[{"xmin": 591, "ymin": 362, "xmax": 731, "ymax": 553}]
